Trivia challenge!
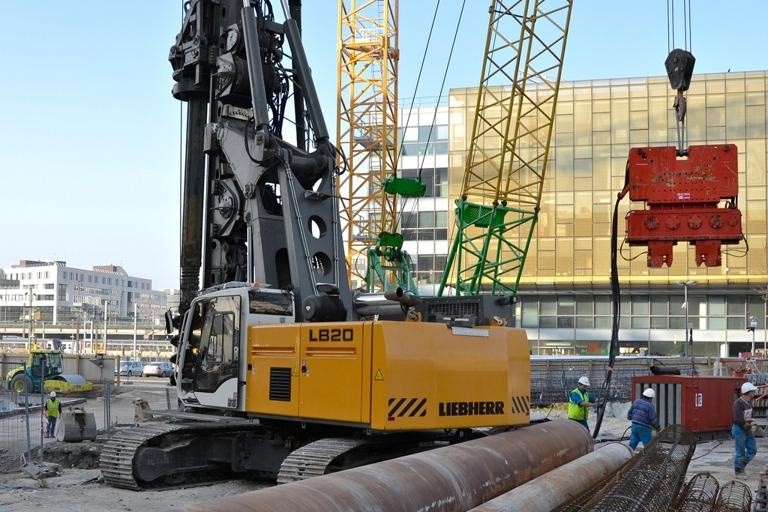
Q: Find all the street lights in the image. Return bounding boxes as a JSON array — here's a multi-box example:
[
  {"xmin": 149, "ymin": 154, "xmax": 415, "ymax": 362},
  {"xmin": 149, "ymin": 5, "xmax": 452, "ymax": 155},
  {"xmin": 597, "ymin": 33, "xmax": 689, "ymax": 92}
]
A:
[
  {"xmin": 749, "ymin": 315, "xmax": 757, "ymax": 355},
  {"xmin": 675, "ymin": 280, "xmax": 696, "ymax": 357}
]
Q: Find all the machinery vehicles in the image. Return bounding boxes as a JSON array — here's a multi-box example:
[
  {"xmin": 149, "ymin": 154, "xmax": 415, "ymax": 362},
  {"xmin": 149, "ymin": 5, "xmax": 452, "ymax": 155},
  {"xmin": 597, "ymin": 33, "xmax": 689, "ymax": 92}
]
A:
[
  {"xmin": 6, "ymin": 349, "xmax": 93, "ymax": 395},
  {"xmin": 99, "ymin": 0, "xmax": 531, "ymax": 491}
]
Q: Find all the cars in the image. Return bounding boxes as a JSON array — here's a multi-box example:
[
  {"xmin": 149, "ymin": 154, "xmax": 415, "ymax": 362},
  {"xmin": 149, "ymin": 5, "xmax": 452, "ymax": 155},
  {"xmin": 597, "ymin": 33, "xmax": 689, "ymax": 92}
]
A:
[
  {"xmin": 114, "ymin": 359, "xmax": 144, "ymax": 376},
  {"xmin": 142, "ymin": 361, "xmax": 177, "ymax": 378}
]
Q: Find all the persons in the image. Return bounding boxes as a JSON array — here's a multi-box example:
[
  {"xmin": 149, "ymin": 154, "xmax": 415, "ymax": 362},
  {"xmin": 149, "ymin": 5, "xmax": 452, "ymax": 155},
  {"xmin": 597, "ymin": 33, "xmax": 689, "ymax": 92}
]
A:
[
  {"xmin": 627, "ymin": 386, "xmax": 664, "ymax": 466},
  {"xmin": 568, "ymin": 375, "xmax": 604, "ymax": 435},
  {"xmin": 43, "ymin": 391, "xmax": 63, "ymax": 438},
  {"xmin": 730, "ymin": 381, "xmax": 760, "ymax": 480}
]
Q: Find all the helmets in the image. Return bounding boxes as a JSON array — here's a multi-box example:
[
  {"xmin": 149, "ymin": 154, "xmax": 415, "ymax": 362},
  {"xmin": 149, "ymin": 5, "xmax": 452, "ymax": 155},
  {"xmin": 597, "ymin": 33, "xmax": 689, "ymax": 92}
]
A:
[
  {"xmin": 578, "ymin": 376, "xmax": 590, "ymax": 386},
  {"xmin": 740, "ymin": 382, "xmax": 757, "ymax": 394},
  {"xmin": 50, "ymin": 392, "xmax": 56, "ymax": 397},
  {"xmin": 643, "ymin": 388, "xmax": 655, "ymax": 397}
]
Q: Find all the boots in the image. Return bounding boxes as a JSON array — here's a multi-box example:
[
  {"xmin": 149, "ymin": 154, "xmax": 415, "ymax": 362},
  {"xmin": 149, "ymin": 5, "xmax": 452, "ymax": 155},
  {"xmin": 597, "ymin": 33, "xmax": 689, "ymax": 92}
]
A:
[
  {"xmin": 46, "ymin": 431, "xmax": 55, "ymax": 438},
  {"xmin": 736, "ymin": 462, "xmax": 748, "ymax": 479}
]
